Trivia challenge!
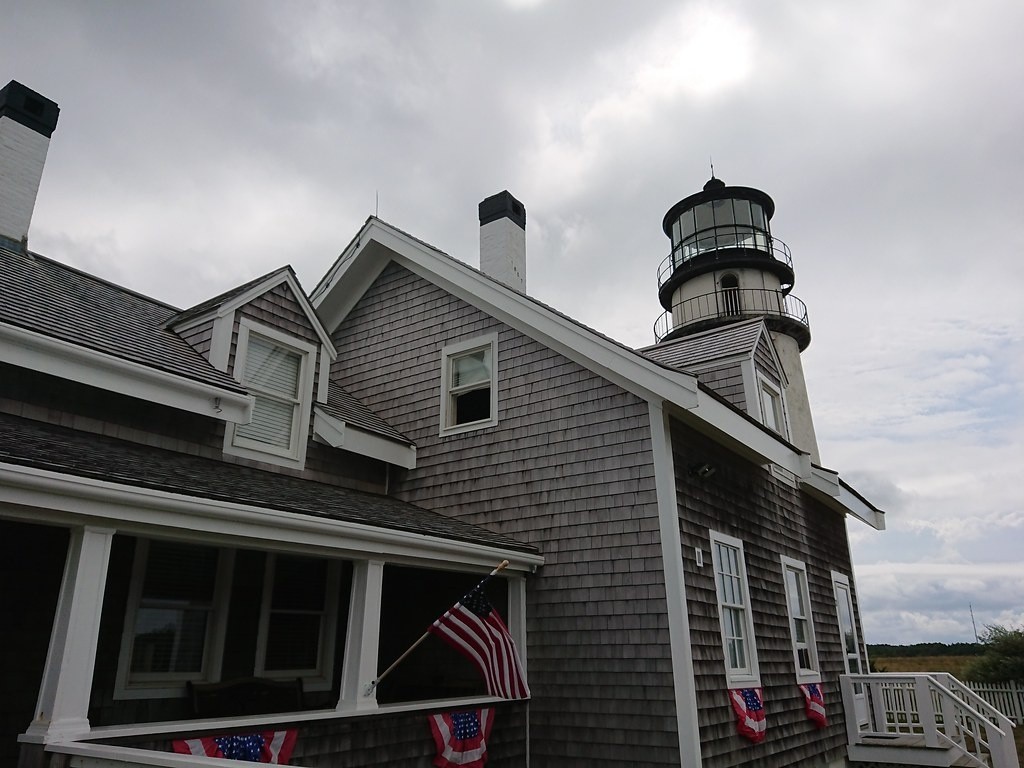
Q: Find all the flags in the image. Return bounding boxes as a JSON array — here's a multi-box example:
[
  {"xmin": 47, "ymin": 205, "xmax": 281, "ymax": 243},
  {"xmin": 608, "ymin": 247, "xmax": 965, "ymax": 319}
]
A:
[
  {"xmin": 430, "ymin": 707, "xmax": 497, "ymax": 767},
  {"xmin": 799, "ymin": 684, "xmax": 829, "ymax": 727},
  {"xmin": 175, "ymin": 730, "xmax": 305, "ymax": 765},
  {"xmin": 433, "ymin": 579, "xmax": 532, "ymax": 699},
  {"xmin": 729, "ymin": 687, "xmax": 767, "ymax": 743}
]
